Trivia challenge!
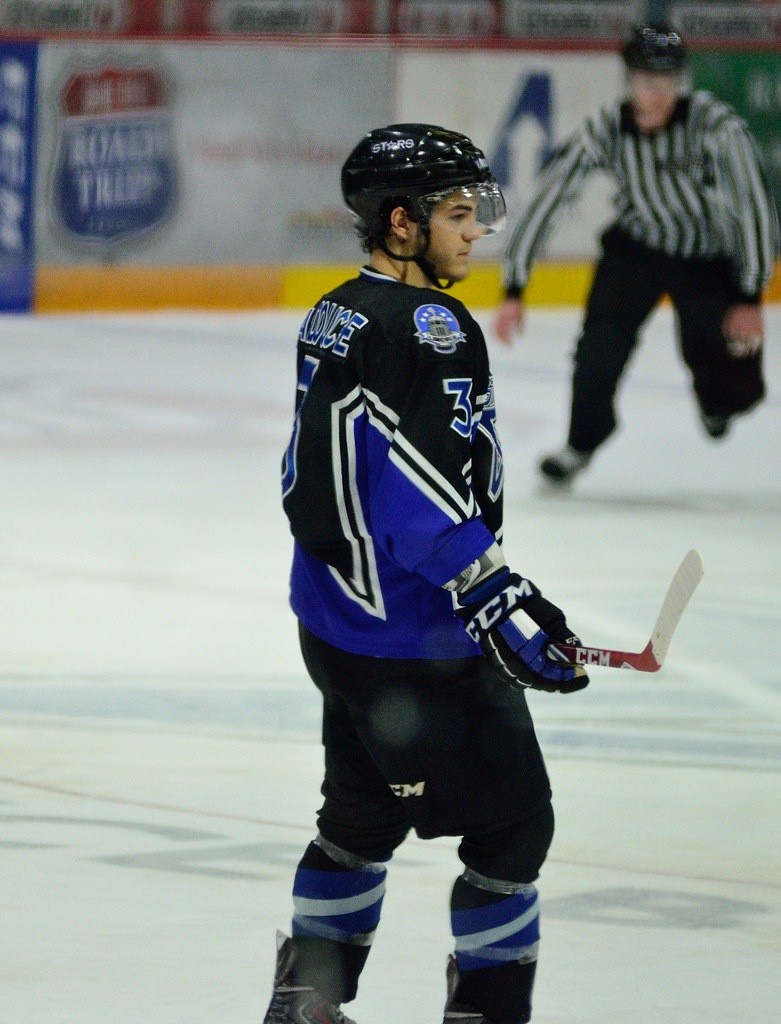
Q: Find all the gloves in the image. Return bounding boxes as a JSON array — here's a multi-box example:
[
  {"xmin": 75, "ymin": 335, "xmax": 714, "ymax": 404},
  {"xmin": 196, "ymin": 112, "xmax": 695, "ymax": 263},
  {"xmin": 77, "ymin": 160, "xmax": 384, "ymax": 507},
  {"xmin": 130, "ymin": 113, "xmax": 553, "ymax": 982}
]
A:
[{"xmin": 414, "ymin": 518, "xmax": 590, "ymax": 694}]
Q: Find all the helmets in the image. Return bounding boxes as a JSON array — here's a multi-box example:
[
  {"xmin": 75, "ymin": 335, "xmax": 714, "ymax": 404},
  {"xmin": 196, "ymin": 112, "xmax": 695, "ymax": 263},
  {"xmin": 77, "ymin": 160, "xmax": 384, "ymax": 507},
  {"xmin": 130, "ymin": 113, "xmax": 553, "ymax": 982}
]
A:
[
  {"xmin": 619, "ymin": 21, "xmax": 689, "ymax": 72},
  {"xmin": 341, "ymin": 123, "xmax": 508, "ymax": 238}
]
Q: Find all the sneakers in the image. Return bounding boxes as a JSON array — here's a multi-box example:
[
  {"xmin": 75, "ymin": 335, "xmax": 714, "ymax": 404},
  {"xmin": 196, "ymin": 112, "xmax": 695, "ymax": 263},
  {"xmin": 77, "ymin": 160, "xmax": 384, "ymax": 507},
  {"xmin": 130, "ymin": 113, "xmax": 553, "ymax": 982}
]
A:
[
  {"xmin": 698, "ymin": 397, "xmax": 737, "ymax": 443},
  {"xmin": 535, "ymin": 441, "xmax": 593, "ymax": 499},
  {"xmin": 262, "ymin": 938, "xmax": 360, "ymax": 1024},
  {"xmin": 442, "ymin": 953, "xmax": 537, "ymax": 1024}
]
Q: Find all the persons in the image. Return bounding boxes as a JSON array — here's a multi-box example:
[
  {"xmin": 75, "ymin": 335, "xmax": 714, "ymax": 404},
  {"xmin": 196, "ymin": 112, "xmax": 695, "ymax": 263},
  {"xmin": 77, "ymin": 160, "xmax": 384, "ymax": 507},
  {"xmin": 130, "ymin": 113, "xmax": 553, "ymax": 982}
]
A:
[
  {"xmin": 263, "ymin": 124, "xmax": 589, "ymax": 1024},
  {"xmin": 496, "ymin": 15, "xmax": 777, "ymax": 480}
]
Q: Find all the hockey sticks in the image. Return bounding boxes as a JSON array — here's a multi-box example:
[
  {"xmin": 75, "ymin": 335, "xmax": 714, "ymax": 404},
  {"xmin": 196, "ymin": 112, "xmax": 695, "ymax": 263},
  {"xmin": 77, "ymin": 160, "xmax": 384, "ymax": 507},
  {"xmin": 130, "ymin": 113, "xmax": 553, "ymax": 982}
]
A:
[{"xmin": 552, "ymin": 547, "xmax": 706, "ymax": 672}]
